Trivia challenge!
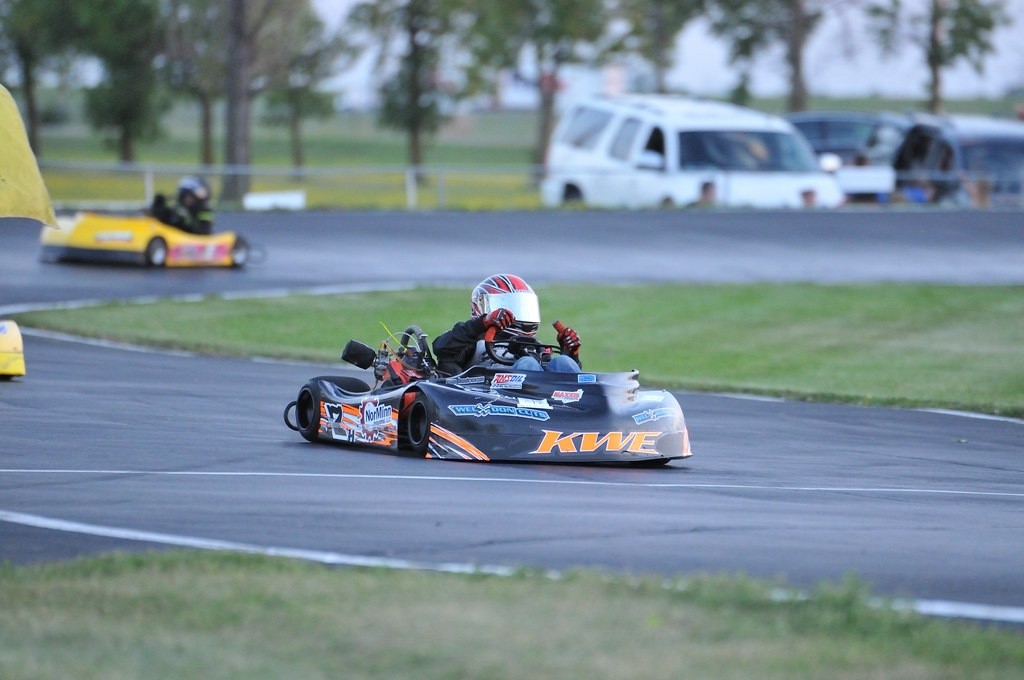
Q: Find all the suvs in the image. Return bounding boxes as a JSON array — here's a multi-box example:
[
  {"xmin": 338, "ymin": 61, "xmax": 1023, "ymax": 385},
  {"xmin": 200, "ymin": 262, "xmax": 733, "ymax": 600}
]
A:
[
  {"xmin": 534, "ymin": 93, "xmax": 851, "ymax": 211},
  {"xmin": 779, "ymin": 109, "xmax": 1024, "ymax": 215}
]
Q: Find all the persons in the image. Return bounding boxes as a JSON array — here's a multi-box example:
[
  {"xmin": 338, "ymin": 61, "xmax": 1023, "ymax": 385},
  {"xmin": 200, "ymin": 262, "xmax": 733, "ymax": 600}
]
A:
[
  {"xmin": 687, "ymin": 146, "xmax": 978, "ymax": 209},
  {"xmin": 168, "ymin": 173, "xmax": 216, "ymax": 238},
  {"xmin": 434, "ymin": 272, "xmax": 583, "ymax": 380}
]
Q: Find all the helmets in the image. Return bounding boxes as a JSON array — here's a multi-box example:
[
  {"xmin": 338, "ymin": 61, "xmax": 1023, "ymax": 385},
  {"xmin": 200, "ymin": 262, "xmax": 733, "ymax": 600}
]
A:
[
  {"xmin": 470, "ymin": 272, "xmax": 541, "ymax": 335},
  {"xmin": 178, "ymin": 178, "xmax": 213, "ymax": 212}
]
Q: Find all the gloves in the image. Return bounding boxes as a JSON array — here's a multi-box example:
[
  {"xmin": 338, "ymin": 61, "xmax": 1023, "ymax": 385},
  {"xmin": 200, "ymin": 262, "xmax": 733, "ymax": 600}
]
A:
[
  {"xmin": 557, "ymin": 327, "xmax": 581, "ymax": 357},
  {"xmin": 483, "ymin": 307, "xmax": 517, "ymax": 330}
]
